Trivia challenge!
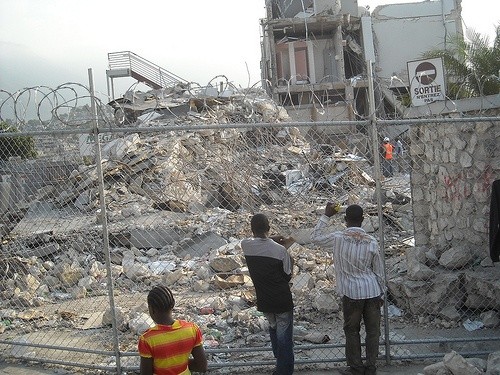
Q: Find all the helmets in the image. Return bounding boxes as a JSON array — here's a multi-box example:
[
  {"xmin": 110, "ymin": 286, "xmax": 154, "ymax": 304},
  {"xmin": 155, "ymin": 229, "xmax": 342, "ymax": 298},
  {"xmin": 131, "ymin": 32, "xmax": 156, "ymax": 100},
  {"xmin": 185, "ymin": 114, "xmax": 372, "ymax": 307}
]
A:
[{"xmin": 383, "ymin": 137, "xmax": 390, "ymax": 142}]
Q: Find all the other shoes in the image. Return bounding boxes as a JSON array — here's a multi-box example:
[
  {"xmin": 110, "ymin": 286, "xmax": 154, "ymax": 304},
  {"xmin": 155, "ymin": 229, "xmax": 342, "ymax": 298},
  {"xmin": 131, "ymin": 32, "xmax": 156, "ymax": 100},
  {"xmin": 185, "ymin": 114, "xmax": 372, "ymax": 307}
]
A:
[{"xmin": 339, "ymin": 366, "xmax": 352, "ymax": 375}]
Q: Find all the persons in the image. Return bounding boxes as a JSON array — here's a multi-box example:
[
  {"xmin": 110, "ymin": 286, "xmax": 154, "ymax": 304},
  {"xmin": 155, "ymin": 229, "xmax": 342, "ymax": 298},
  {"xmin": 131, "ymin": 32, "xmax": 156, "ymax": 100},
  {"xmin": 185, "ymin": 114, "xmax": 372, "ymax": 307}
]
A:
[
  {"xmin": 241, "ymin": 214, "xmax": 295, "ymax": 375},
  {"xmin": 380, "ymin": 137, "xmax": 393, "ymax": 177},
  {"xmin": 394, "ymin": 136, "xmax": 404, "ymax": 173},
  {"xmin": 311, "ymin": 202, "xmax": 386, "ymax": 375},
  {"xmin": 138, "ymin": 286, "xmax": 207, "ymax": 375}
]
